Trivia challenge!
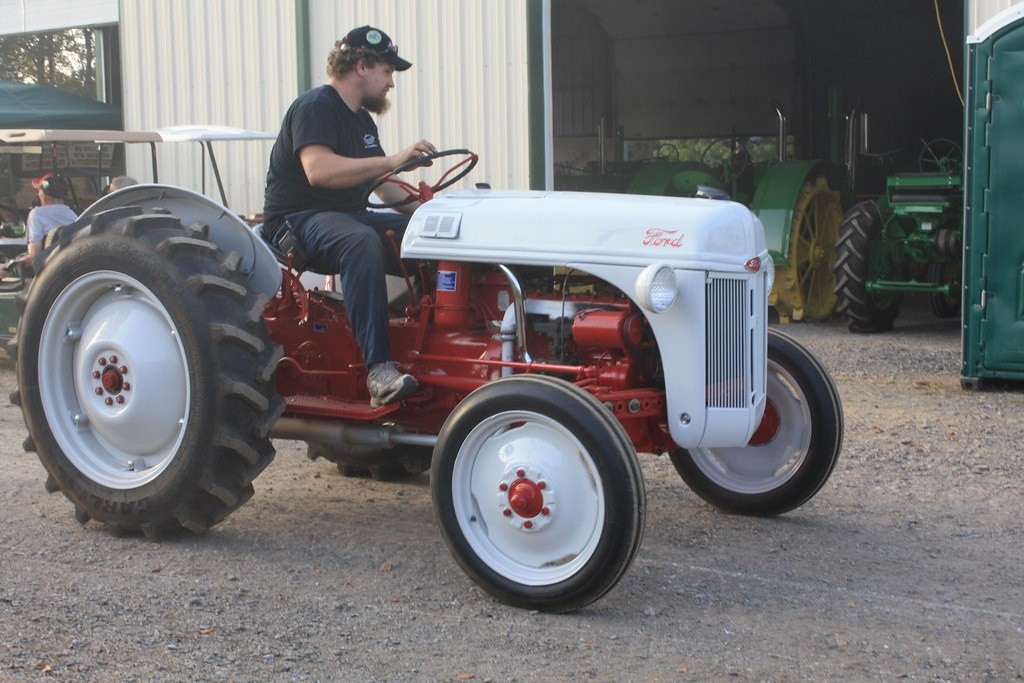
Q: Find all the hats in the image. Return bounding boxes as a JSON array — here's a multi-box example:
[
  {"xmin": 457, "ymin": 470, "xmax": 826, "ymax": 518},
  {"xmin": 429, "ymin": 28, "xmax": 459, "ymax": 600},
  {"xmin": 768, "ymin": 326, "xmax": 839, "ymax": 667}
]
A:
[
  {"xmin": 340, "ymin": 25, "xmax": 413, "ymax": 73},
  {"xmin": 32, "ymin": 171, "xmax": 68, "ymax": 199}
]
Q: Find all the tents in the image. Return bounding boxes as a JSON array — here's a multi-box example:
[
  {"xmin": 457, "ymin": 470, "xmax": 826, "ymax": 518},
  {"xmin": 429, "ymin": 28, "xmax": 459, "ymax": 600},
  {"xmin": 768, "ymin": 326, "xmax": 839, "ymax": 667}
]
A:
[{"xmin": 0, "ymin": 77, "xmax": 122, "ymax": 217}]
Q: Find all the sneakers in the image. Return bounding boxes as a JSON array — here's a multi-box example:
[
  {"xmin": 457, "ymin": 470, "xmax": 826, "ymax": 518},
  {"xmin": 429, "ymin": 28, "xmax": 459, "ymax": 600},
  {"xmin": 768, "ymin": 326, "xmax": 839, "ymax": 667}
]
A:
[{"xmin": 366, "ymin": 359, "xmax": 419, "ymax": 409}]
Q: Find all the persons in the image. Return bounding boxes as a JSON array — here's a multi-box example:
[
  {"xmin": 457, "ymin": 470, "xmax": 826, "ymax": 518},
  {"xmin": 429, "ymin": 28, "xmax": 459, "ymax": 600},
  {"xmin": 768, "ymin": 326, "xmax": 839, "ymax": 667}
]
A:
[
  {"xmin": 105, "ymin": 176, "xmax": 138, "ymax": 194},
  {"xmin": 263, "ymin": 25, "xmax": 438, "ymax": 408},
  {"xmin": 0, "ymin": 172, "xmax": 79, "ymax": 279}
]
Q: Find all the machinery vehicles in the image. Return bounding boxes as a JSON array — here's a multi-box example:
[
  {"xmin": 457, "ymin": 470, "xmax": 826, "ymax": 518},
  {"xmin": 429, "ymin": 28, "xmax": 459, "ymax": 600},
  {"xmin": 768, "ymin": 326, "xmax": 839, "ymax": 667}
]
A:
[
  {"xmin": 554, "ymin": 103, "xmax": 962, "ymax": 334},
  {"xmin": 9, "ymin": 128, "xmax": 846, "ymax": 615}
]
